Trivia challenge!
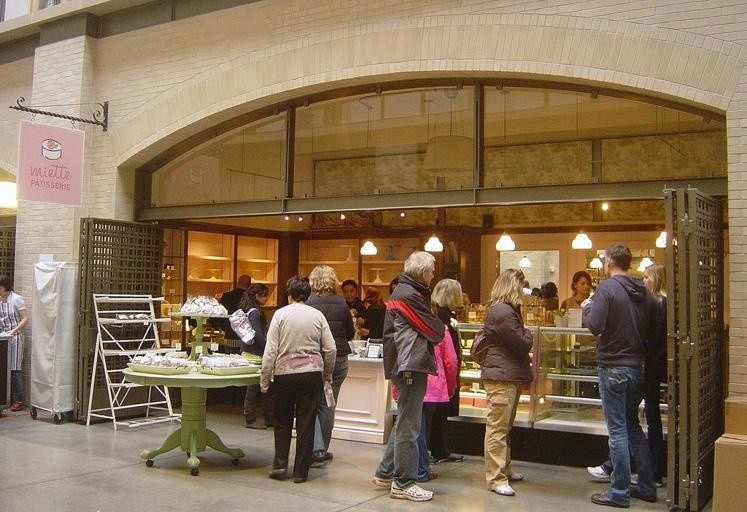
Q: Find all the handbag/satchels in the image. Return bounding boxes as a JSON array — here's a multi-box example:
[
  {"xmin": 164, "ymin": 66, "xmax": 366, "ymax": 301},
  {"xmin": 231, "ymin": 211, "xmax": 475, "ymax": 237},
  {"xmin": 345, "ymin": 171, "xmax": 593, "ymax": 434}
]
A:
[
  {"xmin": 229, "ymin": 308, "xmax": 261, "ymax": 345},
  {"xmin": 470, "ymin": 332, "xmax": 488, "ymax": 365}
]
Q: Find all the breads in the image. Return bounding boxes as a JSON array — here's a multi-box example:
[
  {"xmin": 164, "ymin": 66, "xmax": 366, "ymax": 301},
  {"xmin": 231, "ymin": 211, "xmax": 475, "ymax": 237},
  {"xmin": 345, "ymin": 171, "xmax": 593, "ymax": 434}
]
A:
[
  {"xmin": 181, "ymin": 295, "xmax": 229, "ymax": 315},
  {"xmin": 131, "ymin": 350, "xmax": 263, "ymax": 368}
]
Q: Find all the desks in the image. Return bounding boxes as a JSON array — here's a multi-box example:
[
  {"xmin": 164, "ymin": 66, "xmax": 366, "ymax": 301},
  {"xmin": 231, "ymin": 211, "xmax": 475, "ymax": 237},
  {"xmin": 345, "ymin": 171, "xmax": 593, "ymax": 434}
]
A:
[
  {"xmin": 122, "ymin": 314, "xmax": 274, "ymax": 475},
  {"xmin": 291, "ymin": 356, "xmax": 393, "ymax": 444}
]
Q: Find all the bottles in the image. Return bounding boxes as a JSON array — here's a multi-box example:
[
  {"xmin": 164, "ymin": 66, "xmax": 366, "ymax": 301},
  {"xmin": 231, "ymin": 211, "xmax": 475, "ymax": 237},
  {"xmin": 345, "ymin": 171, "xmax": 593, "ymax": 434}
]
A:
[{"xmin": 323, "ymin": 380, "xmax": 335, "ymax": 407}]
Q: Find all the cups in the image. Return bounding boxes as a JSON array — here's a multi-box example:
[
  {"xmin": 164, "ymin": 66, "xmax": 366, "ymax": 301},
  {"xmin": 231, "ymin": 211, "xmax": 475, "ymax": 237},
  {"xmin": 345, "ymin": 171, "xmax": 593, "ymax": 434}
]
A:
[{"xmin": 573, "ymin": 342, "xmax": 580, "ymax": 349}]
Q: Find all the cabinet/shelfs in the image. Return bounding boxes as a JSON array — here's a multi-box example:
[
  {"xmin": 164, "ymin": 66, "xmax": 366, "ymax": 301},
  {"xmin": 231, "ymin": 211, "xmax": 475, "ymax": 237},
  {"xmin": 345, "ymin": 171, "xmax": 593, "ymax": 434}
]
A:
[
  {"xmin": 391, "ymin": 323, "xmax": 539, "ymax": 464},
  {"xmin": 236, "ymin": 234, "xmax": 280, "ymax": 309},
  {"xmin": 298, "ymin": 238, "xmax": 360, "ymax": 301},
  {"xmin": 358, "ymin": 236, "xmax": 422, "ymax": 307},
  {"xmin": 185, "ymin": 230, "xmax": 235, "ymax": 307},
  {"xmin": 532, "ymin": 327, "xmax": 667, "ymax": 478},
  {"xmin": 86, "ymin": 292, "xmax": 182, "ymax": 429}
]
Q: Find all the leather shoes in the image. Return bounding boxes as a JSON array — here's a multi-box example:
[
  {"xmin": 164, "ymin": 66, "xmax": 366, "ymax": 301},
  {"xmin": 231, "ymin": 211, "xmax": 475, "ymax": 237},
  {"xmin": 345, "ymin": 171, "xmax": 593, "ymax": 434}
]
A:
[
  {"xmin": 631, "ymin": 488, "xmax": 656, "ymax": 502},
  {"xmin": 591, "ymin": 493, "xmax": 630, "ymax": 508}
]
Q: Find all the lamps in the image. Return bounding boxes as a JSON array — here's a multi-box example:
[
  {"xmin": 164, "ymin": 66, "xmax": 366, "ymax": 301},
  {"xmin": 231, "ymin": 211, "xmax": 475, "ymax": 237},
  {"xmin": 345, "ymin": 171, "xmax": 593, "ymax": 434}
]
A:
[
  {"xmin": 590, "ymin": 258, "xmax": 603, "ymax": 269},
  {"xmin": 638, "ymin": 256, "xmax": 653, "ymax": 272},
  {"xmin": 519, "ymin": 256, "xmax": 531, "ymax": 268},
  {"xmin": 424, "ymin": 235, "xmax": 443, "ymax": 253},
  {"xmin": 360, "ymin": 241, "xmax": 378, "ymax": 255},
  {"xmin": 655, "ymin": 229, "xmax": 667, "ymax": 249},
  {"xmin": 496, "ymin": 232, "xmax": 515, "ymax": 251},
  {"xmin": 572, "ymin": 232, "xmax": 592, "ymax": 250}
]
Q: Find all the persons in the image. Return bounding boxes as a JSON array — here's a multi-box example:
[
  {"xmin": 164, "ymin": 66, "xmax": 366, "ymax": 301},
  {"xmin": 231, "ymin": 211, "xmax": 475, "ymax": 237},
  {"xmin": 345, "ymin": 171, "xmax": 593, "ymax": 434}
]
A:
[
  {"xmin": 362, "ymin": 285, "xmax": 386, "ymax": 341},
  {"xmin": 256, "ymin": 274, "xmax": 338, "ymax": 483},
  {"xmin": 340, "ymin": 279, "xmax": 367, "ymax": 339},
  {"xmin": 0, "ymin": 273, "xmax": 28, "ymax": 412},
  {"xmin": 214, "ymin": 273, "xmax": 251, "ymax": 406},
  {"xmin": 370, "ymin": 251, "xmax": 445, "ymax": 501},
  {"xmin": 388, "ymin": 278, "xmax": 398, "ymax": 294},
  {"xmin": 236, "ymin": 282, "xmax": 273, "ymax": 430},
  {"xmin": 578, "ymin": 244, "xmax": 660, "ymax": 507},
  {"xmin": 423, "ymin": 278, "xmax": 465, "ymax": 464},
  {"xmin": 479, "ymin": 266, "xmax": 533, "ymax": 496},
  {"xmin": 303, "ymin": 265, "xmax": 357, "ymax": 468},
  {"xmin": 416, "ymin": 323, "xmax": 458, "ymax": 482},
  {"xmin": 584, "ymin": 260, "xmax": 667, "ymax": 490},
  {"xmin": 522, "ymin": 280, "xmax": 558, "ymax": 311},
  {"xmin": 559, "ymin": 271, "xmax": 591, "ymax": 312}
]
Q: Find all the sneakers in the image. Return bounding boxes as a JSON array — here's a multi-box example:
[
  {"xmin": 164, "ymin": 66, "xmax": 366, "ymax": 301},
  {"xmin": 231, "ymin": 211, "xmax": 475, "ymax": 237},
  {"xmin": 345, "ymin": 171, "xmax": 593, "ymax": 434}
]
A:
[
  {"xmin": 371, "ymin": 454, "xmax": 464, "ymax": 502},
  {"xmin": 246, "ymin": 423, "xmax": 267, "ymax": 429},
  {"xmin": 11, "ymin": 401, "xmax": 24, "ymax": 411},
  {"xmin": 586, "ymin": 466, "xmax": 610, "ymax": 479},
  {"xmin": 493, "ymin": 485, "xmax": 515, "ymax": 496},
  {"xmin": 269, "ymin": 452, "xmax": 332, "ymax": 483},
  {"xmin": 631, "ymin": 474, "xmax": 662, "ymax": 487},
  {"xmin": 509, "ymin": 472, "xmax": 523, "ymax": 481}
]
